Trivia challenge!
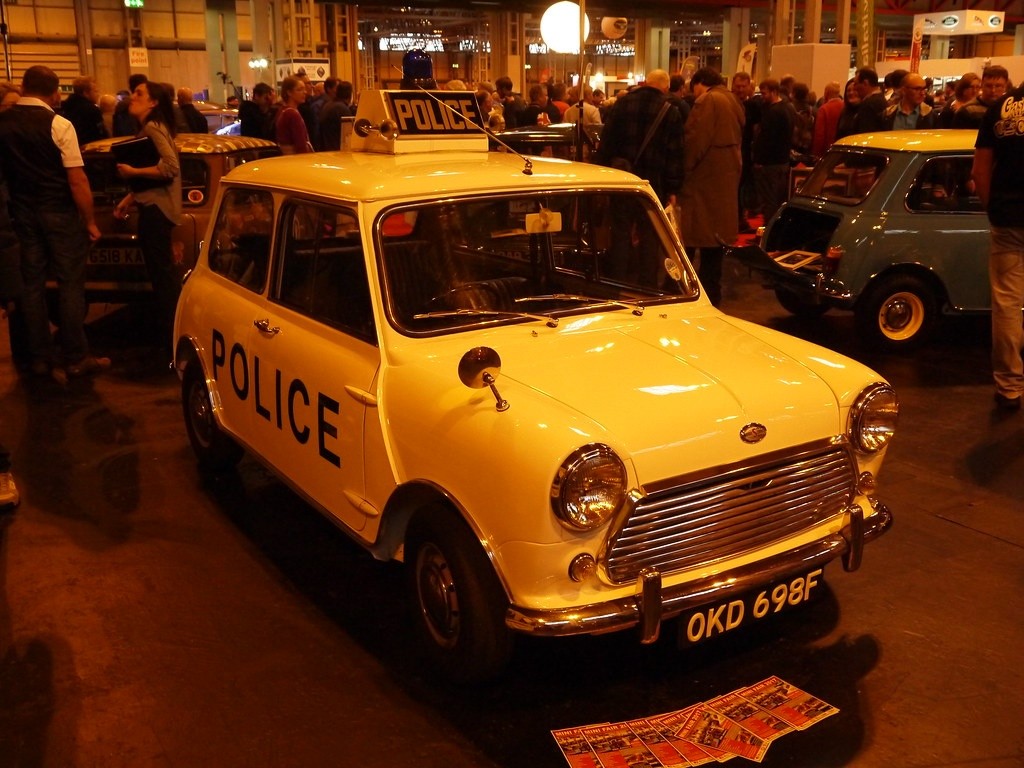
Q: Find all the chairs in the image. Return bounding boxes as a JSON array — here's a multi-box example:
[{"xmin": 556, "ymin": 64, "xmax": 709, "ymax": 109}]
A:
[{"xmin": 450, "ymin": 277, "xmax": 548, "ymax": 319}]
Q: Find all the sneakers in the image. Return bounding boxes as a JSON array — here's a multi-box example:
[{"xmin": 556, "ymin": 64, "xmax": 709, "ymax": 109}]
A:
[{"xmin": 994, "ymin": 392, "xmax": 1021, "ymax": 411}]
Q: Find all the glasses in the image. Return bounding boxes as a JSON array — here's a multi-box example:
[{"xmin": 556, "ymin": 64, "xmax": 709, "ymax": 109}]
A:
[
  {"xmin": 985, "ymin": 84, "xmax": 1007, "ymax": 90},
  {"xmin": 967, "ymin": 85, "xmax": 980, "ymax": 90},
  {"xmin": 907, "ymin": 87, "xmax": 928, "ymax": 94}
]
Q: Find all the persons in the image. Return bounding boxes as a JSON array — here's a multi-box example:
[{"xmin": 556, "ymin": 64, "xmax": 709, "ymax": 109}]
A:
[
  {"xmin": 442, "ymin": 64, "xmax": 1024, "ymax": 255},
  {"xmin": 0, "ymin": 66, "xmax": 101, "ymax": 405},
  {"xmin": 0, "ymin": 69, "xmax": 359, "ymax": 317},
  {"xmin": 682, "ymin": 66, "xmax": 745, "ymax": 309},
  {"xmin": 973, "ymin": 81, "xmax": 1024, "ymax": 412},
  {"xmin": 755, "ymin": 79, "xmax": 794, "ymax": 226},
  {"xmin": 114, "ymin": 81, "xmax": 183, "ymax": 373},
  {"xmin": 598, "ymin": 69, "xmax": 689, "ymax": 288}
]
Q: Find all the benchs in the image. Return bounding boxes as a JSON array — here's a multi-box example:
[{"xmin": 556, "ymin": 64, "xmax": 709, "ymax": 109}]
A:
[{"xmin": 286, "ymin": 240, "xmax": 440, "ymax": 325}]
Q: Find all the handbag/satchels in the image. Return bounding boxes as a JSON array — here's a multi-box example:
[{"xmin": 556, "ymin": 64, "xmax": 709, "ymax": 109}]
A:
[{"xmin": 609, "ymin": 158, "xmax": 632, "ymax": 172}]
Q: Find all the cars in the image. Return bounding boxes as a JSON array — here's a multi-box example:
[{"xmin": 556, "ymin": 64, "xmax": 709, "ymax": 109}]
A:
[
  {"xmin": 76, "ymin": 133, "xmax": 333, "ymax": 327},
  {"xmin": 749, "ymin": 128, "xmax": 993, "ymax": 349},
  {"xmin": 172, "ymin": 49, "xmax": 899, "ymax": 684},
  {"xmin": 453, "ymin": 108, "xmax": 646, "ymax": 276}
]
